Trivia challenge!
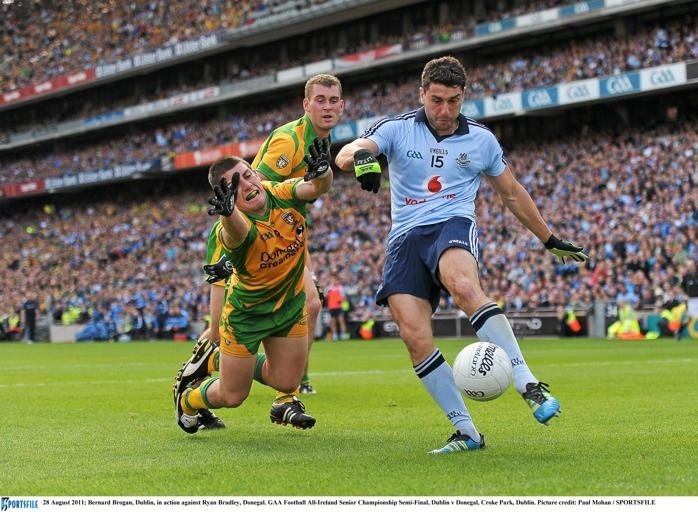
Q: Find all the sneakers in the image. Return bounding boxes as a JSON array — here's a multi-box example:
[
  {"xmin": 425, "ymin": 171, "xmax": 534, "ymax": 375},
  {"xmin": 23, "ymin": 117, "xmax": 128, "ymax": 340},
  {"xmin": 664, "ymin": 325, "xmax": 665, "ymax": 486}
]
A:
[
  {"xmin": 174, "ymin": 387, "xmax": 203, "ymax": 434},
  {"xmin": 299, "ymin": 384, "xmax": 316, "ymax": 394},
  {"xmin": 522, "ymin": 382, "xmax": 562, "ymax": 426},
  {"xmin": 270, "ymin": 396, "xmax": 316, "ymax": 430},
  {"xmin": 196, "ymin": 408, "xmax": 225, "ymax": 430},
  {"xmin": 172, "ymin": 337, "xmax": 219, "ymax": 393},
  {"xmin": 427, "ymin": 429, "xmax": 486, "ymax": 455}
]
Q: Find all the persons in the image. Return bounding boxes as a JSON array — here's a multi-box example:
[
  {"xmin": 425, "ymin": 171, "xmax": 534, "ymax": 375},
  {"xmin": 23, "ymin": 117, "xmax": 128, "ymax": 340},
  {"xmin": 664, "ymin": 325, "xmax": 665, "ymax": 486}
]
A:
[
  {"xmin": 0, "ymin": 1, "xmax": 697, "ymax": 340},
  {"xmin": 335, "ymin": 56, "xmax": 592, "ymax": 455},
  {"xmin": 171, "ymin": 138, "xmax": 335, "ymax": 432},
  {"xmin": 204, "ymin": 74, "xmax": 344, "ymax": 431}
]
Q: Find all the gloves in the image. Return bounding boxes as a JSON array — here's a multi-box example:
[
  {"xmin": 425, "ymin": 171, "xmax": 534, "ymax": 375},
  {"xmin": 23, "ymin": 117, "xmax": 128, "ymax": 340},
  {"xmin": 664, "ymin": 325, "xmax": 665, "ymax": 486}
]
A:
[
  {"xmin": 353, "ymin": 149, "xmax": 382, "ymax": 194},
  {"xmin": 302, "ymin": 136, "xmax": 332, "ymax": 182},
  {"xmin": 207, "ymin": 172, "xmax": 240, "ymax": 217},
  {"xmin": 543, "ymin": 233, "xmax": 590, "ymax": 265},
  {"xmin": 203, "ymin": 254, "xmax": 233, "ymax": 285}
]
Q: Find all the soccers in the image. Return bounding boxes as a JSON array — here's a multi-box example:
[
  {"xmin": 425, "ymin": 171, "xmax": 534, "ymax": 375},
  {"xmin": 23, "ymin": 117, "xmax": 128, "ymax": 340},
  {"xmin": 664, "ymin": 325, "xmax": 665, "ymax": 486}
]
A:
[{"xmin": 453, "ymin": 342, "xmax": 513, "ymax": 401}]
